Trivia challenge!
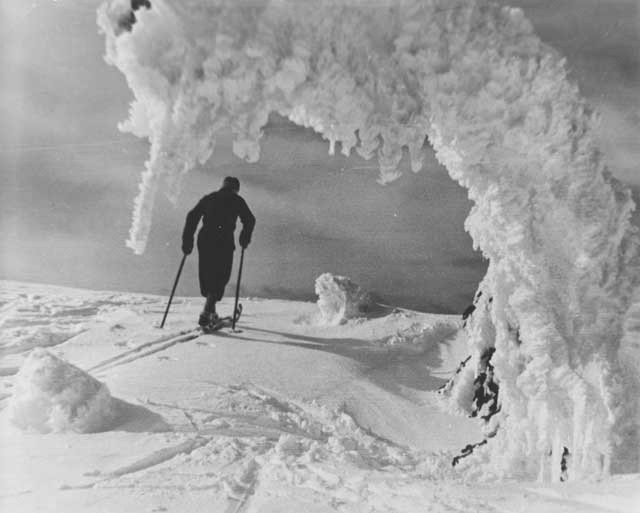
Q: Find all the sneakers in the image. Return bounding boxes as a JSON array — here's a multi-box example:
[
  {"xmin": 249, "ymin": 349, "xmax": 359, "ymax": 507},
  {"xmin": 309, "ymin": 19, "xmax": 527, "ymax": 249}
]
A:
[{"xmin": 198, "ymin": 312, "xmax": 216, "ymax": 325}]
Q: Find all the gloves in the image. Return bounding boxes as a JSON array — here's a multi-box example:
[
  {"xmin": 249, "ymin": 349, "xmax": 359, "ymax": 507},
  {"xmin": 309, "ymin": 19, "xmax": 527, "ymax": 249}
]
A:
[
  {"xmin": 239, "ymin": 232, "xmax": 251, "ymax": 246},
  {"xmin": 182, "ymin": 239, "xmax": 193, "ymax": 254}
]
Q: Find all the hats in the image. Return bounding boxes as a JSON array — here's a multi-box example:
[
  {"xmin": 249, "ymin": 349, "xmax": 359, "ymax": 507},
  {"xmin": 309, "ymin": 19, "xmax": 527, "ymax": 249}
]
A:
[{"xmin": 224, "ymin": 176, "xmax": 239, "ymax": 191}]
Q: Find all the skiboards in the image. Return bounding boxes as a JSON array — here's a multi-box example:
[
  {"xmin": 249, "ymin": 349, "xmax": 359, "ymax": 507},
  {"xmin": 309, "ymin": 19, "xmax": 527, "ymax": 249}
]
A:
[{"xmin": 180, "ymin": 303, "xmax": 242, "ymax": 342}]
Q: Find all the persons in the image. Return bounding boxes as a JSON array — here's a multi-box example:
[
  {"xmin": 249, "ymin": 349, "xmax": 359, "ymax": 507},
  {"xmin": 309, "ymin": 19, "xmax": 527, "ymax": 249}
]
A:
[{"xmin": 181, "ymin": 176, "xmax": 256, "ymax": 326}]
